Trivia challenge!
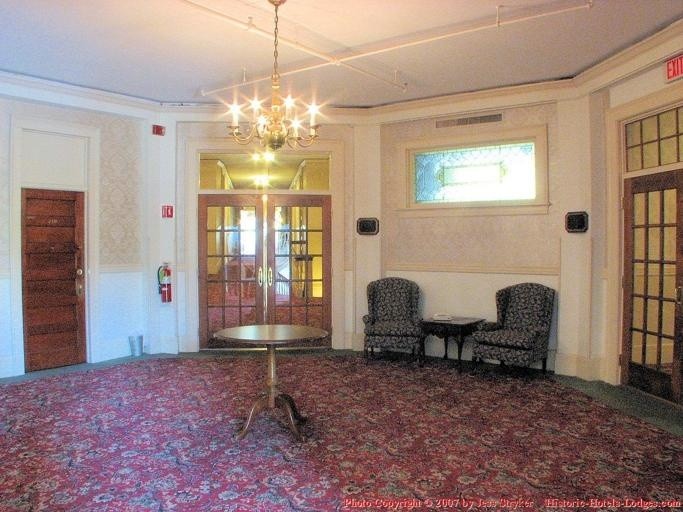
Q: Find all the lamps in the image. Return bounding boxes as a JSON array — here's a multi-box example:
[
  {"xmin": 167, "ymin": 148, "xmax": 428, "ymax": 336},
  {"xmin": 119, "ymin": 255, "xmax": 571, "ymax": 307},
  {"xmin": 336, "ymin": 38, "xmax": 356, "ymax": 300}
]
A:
[{"xmin": 226, "ymin": 0, "xmax": 322, "ymax": 152}]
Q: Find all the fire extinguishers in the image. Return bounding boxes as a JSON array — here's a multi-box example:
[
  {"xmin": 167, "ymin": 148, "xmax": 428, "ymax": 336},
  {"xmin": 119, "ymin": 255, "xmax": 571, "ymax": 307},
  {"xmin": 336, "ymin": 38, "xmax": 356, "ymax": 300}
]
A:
[{"xmin": 156, "ymin": 260, "xmax": 171, "ymax": 304}]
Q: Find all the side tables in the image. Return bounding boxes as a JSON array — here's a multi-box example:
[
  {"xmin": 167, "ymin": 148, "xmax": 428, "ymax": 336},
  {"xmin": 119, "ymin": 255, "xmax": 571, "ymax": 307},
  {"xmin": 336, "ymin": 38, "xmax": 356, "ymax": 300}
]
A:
[{"xmin": 419, "ymin": 316, "xmax": 486, "ymax": 373}]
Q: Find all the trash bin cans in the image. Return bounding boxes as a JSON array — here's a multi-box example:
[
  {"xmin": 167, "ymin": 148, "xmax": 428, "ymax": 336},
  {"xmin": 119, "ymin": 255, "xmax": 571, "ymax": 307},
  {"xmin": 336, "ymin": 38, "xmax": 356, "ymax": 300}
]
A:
[{"xmin": 128, "ymin": 334, "xmax": 144, "ymax": 357}]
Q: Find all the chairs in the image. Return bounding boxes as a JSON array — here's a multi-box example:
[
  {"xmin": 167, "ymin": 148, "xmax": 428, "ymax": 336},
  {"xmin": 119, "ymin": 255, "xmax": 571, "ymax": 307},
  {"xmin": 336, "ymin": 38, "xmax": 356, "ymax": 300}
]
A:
[
  {"xmin": 358, "ymin": 276, "xmax": 426, "ymax": 369},
  {"xmin": 469, "ymin": 281, "xmax": 556, "ymax": 380}
]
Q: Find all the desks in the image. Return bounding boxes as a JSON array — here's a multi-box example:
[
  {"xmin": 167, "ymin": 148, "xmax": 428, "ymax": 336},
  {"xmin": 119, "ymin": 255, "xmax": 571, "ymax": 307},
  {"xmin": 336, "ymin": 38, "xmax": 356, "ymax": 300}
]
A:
[{"xmin": 211, "ymin": 323, "xmax": 330, "ymax": 441}]
